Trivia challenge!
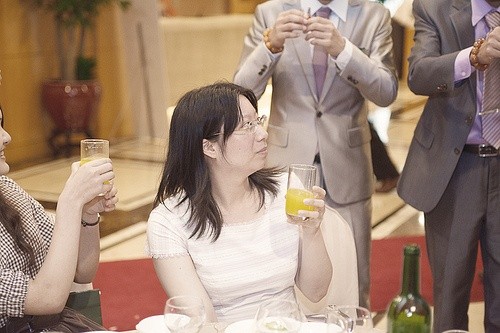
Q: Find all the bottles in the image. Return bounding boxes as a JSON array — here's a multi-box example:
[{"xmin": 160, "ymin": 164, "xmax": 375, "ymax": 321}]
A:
[{"xmin": 387, "ymin": 243, "xmax": 432, "ymax": 333}]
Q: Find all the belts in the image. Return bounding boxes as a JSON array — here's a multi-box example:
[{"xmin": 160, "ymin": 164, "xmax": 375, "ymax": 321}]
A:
[{"xmin": 462, "ymin": 142, "xmax": 500, "ymax": 157}]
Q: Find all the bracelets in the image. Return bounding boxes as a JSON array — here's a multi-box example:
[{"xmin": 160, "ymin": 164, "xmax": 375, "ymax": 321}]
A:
[
  {"xmin": 80, "ymin": 213, "xmax": 101, "ymax": 227},
  {"xmin": 262, "ymin": 28, "xmax": 283, "ymax": 53},
  {"xmin": 471, "ymin": 39, "xmax": 490, "ymax": 71}
]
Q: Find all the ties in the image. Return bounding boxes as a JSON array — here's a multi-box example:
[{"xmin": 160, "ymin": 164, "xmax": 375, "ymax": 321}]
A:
[
  {"xmin": 312, "ymin": 7, "xmax": 332, "ymax": 99},
  {"xmin": 481, "ymin": 12, "xmax": 500, "ymax": 150}
]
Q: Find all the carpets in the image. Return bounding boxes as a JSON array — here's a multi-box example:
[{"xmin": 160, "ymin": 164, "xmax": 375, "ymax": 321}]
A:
[{"xmin": 92, "ymin": 233, "xmax": 487, "ymax": 332}]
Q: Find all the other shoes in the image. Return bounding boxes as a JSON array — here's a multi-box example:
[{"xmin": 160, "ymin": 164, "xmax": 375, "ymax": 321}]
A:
[{"xmin": 375, "ymin": 175, "xmax": 401, "ymax": 193}]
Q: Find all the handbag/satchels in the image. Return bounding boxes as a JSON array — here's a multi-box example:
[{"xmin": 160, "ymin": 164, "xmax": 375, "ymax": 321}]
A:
[{"xmin": 5, "ymin": 304, "xmax": 108, "ymax": 333}]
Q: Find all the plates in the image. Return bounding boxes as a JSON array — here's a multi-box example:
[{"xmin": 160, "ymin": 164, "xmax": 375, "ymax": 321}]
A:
[
  {"xmin": 224, "ymin": 316, "xmax": 347, "ymax": 333},
  {"xmin": 133, "ymin": 313, "xmax": 192, "ymax": 333}
]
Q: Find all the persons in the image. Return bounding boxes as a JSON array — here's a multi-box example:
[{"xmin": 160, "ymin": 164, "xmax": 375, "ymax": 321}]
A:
[
  {"xmin": 240, "ymin": 0, "xmax": 500, "ymax": 333},
  {"xmin": 148, "ymin": 81, "xmax": 333, "ymax": 326},
  {"xmin": 0, "ymin": 108, "xmax": 121, "ymax": 332}
]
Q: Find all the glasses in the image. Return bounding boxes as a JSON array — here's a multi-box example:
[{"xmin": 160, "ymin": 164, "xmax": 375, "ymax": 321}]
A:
[{"xmin": 209, "ymin": 115, "xmax": 267, "ymax": 137}]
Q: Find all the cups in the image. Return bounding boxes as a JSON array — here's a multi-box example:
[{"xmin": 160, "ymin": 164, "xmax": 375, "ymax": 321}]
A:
[
  {"xmin": 285, "ymin": 164, "xmax": 317, "ymax": 222},
  {"xmin": 163, "ymin": 295, "xmax": 206, "ymax": 333},
  {"xmin": 80, "ymin": 138, "xmax": 110, "ymax": 197},
  {"xmin": 325, "ymin": 305, "xmax": 374, "ymax": 333},
  {"xmin": 255, "ymin": 300, "xmax": 302, "ymax": 333}
]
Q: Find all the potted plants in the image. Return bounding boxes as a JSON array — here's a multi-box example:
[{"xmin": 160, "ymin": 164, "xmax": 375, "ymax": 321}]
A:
[{"xmin": 23, "ymin": 0, "xmax": 130, "ymax": 130}]
